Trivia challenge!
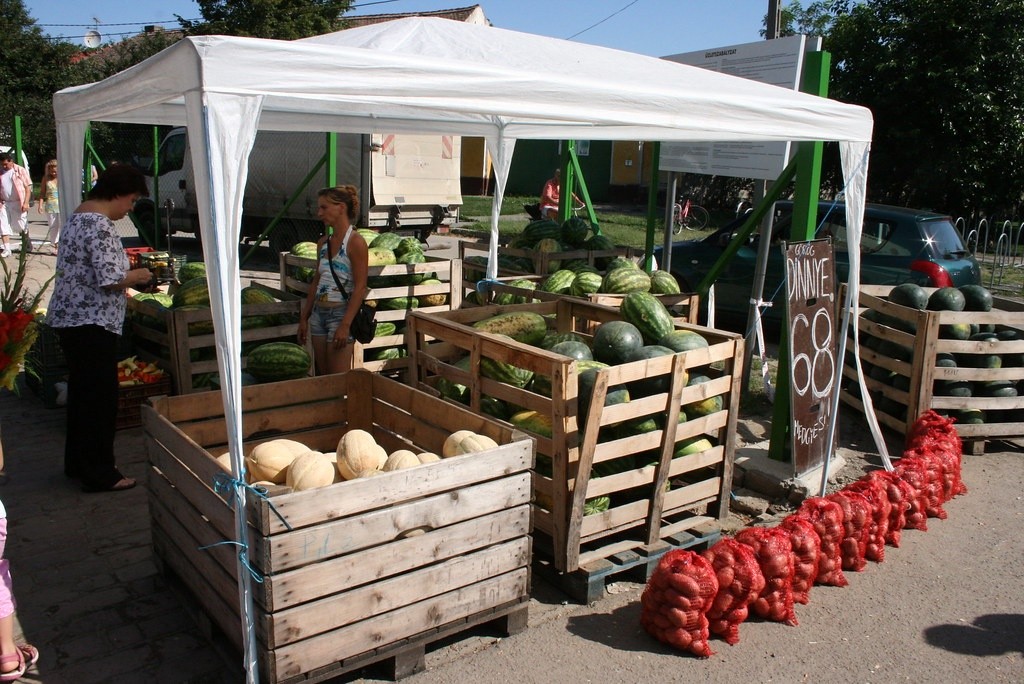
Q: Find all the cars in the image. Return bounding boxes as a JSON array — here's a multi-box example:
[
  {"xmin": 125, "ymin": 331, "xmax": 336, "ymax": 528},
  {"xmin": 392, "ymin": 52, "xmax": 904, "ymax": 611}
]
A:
[{"xmin": 636, "ymin": 201, "xmax": 981, "ymax": 340}]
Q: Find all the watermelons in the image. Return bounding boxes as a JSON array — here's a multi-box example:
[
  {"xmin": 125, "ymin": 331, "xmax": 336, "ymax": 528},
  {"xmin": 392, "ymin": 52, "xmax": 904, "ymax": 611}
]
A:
[
  {"xmin": 435, "ymin": 291, "xmax": 722, "ymax": 516},
  {"xmin": 466, "ymin": 217, "xmax": 681, "ymax": 306},
  {"xmin": 845, "ymin": 282, "xmax": 1024, "ymax": 425},
  {"xmin": 128, "ymin": 261, "xmax": 310, "ymax": 389},
  {"xmin": 284, "ymin": 229, "xmax": 447, "ymax": 360}
]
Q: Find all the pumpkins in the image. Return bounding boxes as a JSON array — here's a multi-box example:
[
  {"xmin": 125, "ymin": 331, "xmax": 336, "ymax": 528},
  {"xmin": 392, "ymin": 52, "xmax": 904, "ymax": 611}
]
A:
[{"xmin": 208, "ymin": 428, "xmax": 499, "ymax": 500}]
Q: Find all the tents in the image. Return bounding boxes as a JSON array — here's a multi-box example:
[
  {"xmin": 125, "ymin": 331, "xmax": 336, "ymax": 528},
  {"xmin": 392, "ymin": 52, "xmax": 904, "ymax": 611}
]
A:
[{"xmin": 53, "ymin": 17, "xmax": 893, "ymax": 684}]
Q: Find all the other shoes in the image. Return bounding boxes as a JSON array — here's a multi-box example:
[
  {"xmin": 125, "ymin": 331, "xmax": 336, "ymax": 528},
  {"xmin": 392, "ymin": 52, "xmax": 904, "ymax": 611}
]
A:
[
  {"xmin": 51, "ymin": 250, "xmax": 57, "ymax": 256},
  {"xmin": 1, "ymin": 249, "xmax": 11, "ymax": 257}
]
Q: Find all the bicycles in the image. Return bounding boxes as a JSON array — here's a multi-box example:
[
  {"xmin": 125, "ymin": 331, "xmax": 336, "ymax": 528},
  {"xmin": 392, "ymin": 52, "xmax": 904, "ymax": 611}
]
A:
[
  {"xmin": 526, "ymin": 203, "xmax": 586, "ymax": 223},
  {"xmin": 672, "ymin": 189, "xmax": 709, "ymax": 236}
]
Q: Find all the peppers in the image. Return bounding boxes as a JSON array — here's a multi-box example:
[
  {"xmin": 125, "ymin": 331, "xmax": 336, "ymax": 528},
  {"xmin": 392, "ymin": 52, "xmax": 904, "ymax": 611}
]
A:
[{"xmin": 117, "ymin": 355, "xmax": 166, "ymax": 387}]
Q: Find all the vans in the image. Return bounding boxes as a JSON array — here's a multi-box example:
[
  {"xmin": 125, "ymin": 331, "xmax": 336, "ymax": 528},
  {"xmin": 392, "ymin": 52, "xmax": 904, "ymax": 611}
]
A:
[{"xmin": 0, "ymin": 146, "xmax": 35, "ymax": 207}]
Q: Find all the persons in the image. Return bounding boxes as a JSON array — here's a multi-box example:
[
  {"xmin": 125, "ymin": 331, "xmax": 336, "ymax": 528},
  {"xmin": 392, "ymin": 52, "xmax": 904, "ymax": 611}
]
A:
[
  {"xmin": 0, "ymin": 443, "xmax": 39, "ymax": 681},
  {"xmin": 82, "ymin": 164, "xmax": 98, "ymax": 189},
  {"xmin": 297, "ymin": 186, "xmax": 368, "ymax": 376},
  {"xmin": 0, "ymin": 153, "xmax": 32, "ymax": 258},
  {"xmin": 38, "ymin": 160, "xmax": 60, "ymax": 256},
  {"xmin": 45, "ymin": 166, "xmax": 160, "ymax": 492},
  {"xmin": 541, "ymin": 168, "xmax": 584, "ymax": 219}
]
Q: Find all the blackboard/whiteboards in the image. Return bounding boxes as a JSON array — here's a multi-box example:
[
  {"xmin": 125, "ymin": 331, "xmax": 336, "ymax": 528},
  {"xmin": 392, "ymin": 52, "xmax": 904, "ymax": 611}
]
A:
[{"xmin": 780, "ymin": 234, "xmax": 840, "ymax": 477}]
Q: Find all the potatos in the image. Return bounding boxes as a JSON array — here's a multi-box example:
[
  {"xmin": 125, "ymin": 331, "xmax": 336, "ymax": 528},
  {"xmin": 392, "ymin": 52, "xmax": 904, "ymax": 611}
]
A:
[{"xmin": 641, "ymin": 419, "xmax": 962, "ymax": 656}]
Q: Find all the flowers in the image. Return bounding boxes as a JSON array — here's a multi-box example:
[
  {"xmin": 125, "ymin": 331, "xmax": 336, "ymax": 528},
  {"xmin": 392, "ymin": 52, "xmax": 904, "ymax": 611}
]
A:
[{"xmin": 0, "ymin": 230, "xmax": 56, "ymax": 392}]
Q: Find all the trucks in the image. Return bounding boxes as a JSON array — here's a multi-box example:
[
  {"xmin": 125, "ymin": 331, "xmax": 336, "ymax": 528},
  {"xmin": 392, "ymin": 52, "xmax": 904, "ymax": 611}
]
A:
[{"xmin": 133, "ymin": 128, "xmax": 464, "ymax": 248}]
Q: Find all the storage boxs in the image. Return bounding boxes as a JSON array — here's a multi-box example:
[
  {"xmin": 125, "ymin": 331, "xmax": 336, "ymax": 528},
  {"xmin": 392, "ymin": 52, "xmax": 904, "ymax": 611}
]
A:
[
  {"xmin": 24, "ymin": 247, "xmax": 745, "ymax": 684},
  {"xmin": 837, "ymin": 283, "xmax": 1024, "ymax": 455}
]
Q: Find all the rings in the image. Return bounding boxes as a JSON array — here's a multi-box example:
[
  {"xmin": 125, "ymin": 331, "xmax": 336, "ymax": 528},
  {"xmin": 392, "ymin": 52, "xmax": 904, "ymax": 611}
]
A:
[{"xmin": 335, "ymin": 340, "xmax": 337, "ymax": 342}]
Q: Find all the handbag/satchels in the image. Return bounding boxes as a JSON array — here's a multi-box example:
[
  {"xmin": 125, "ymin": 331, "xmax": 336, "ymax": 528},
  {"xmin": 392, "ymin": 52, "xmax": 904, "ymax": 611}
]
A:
[{"xmin": 350, "ymin": 304, "xmax": 378, "ymax": 345}]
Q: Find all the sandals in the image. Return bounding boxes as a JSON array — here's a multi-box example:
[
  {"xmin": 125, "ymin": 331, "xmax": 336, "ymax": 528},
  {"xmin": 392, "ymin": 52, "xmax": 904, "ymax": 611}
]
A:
[
  {"xmin": 86, "ymin": 476, "xmax": 136, "ymax": 493},
  {"xmin": 0, "ymin": 643, "xmax": 39, "ymax": 682}
]
